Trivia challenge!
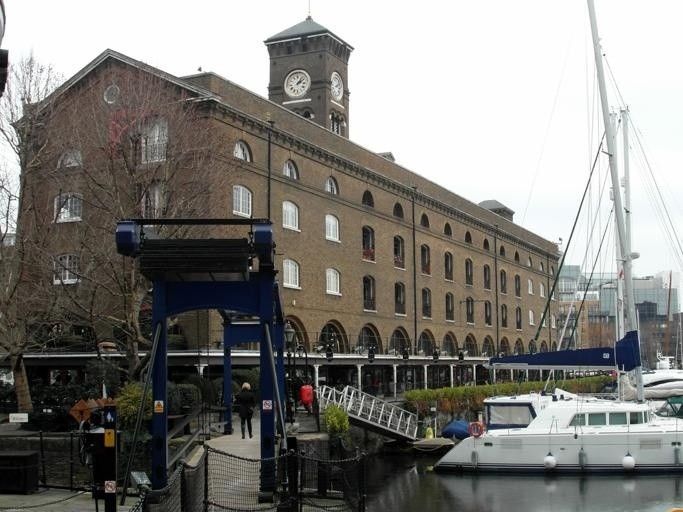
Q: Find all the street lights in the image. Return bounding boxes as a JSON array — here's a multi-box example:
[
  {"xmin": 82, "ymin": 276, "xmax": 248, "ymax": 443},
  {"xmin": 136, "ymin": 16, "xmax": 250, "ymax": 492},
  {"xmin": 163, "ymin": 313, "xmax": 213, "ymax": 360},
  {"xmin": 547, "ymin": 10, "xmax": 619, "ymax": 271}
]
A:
[{"xmin": 281, "ymin": 320, "xmax": 297, "ymax": 424}]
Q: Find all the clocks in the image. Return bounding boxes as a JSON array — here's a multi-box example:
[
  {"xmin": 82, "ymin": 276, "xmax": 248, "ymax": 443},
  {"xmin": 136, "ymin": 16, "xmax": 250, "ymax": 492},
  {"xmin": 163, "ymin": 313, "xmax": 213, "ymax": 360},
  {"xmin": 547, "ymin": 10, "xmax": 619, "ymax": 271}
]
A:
[
  {"xmin": 285, "ymin": 69, "xmax": 310, "ymax": 98},
  {"xmin": 330, "ymin": 71, "xmax": 344, "ymax": 100}
]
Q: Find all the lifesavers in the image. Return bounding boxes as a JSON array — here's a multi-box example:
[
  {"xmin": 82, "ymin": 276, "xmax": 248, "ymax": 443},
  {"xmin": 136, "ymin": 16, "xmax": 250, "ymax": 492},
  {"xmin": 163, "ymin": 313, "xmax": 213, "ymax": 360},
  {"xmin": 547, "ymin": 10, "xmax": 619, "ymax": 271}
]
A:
[{"xmin": 469, "ymin": 422, "xmax": 483, "ymax": 437}]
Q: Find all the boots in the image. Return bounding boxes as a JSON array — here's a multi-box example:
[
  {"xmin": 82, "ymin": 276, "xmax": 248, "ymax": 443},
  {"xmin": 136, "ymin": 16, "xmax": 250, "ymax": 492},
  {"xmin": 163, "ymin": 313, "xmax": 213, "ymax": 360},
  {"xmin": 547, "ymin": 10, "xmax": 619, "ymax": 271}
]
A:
[
  {"xmin": 241, "ymin": 424, "xmax": 245, "ymax": 439},
  {"xmin": 248, "ymin": 424, "xmax": 253, "ymax": 438}
]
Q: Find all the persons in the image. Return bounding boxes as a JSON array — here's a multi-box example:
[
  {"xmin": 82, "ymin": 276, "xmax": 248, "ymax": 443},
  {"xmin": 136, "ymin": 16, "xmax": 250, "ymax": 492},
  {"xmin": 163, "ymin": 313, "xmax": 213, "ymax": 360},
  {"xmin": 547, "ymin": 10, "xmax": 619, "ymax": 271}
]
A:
[{"xmin": 239, "ymin": 382, "xmax": 257, "ymax": 439}]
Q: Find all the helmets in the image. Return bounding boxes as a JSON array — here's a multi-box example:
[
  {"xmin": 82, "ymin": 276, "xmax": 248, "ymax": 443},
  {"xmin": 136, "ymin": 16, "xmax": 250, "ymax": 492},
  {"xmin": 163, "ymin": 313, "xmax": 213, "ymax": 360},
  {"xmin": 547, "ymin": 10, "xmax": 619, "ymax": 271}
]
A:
[{"xmin": 242, "ymin": 382, "xmax": 250, "ymax": 390}]
